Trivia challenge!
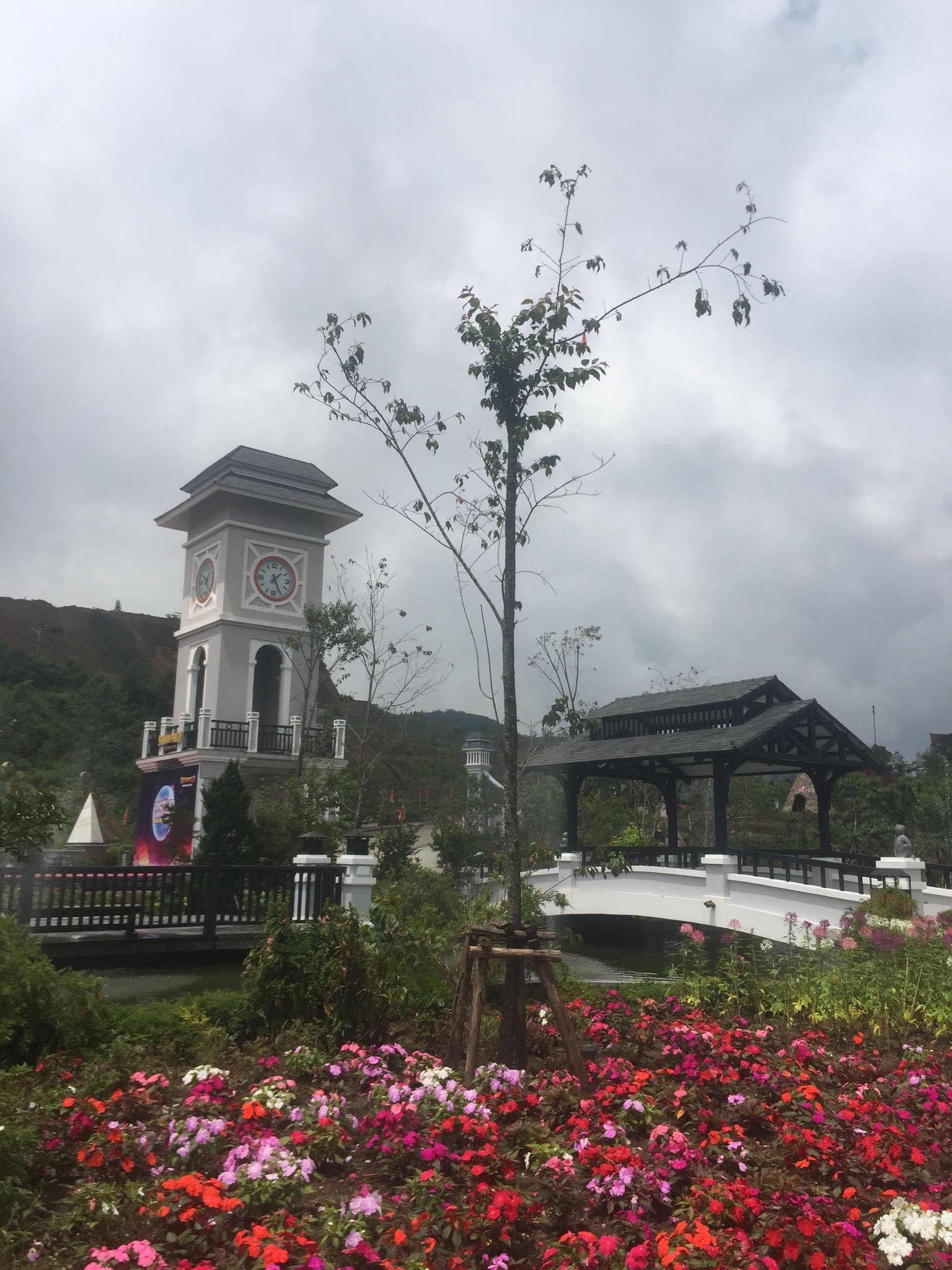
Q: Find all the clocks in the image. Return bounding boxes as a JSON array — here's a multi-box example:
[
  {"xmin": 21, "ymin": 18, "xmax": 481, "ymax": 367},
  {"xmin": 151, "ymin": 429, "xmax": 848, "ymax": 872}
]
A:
[
  {"xmin": 196, "ymin": 558, "xmax": 214, "ymax": 602},
  {"xmin": 254, "ymin": 556, "xmax": 296, "ymax": 602}
]
